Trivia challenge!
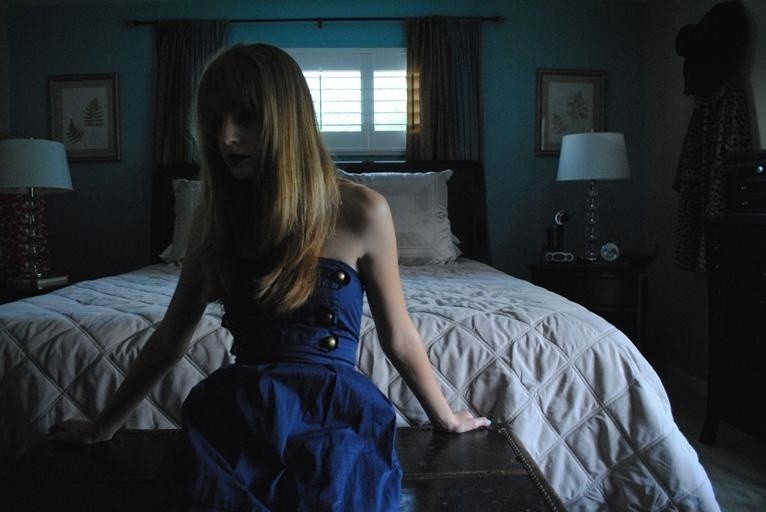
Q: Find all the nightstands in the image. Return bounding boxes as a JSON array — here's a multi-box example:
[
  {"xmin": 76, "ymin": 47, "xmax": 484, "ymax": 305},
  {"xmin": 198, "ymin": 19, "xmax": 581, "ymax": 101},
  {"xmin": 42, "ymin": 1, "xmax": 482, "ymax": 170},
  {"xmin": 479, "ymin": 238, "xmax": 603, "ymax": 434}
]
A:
[{"xmin": 527, "ymin": 253, "xmax": 657, "ymax": 342}]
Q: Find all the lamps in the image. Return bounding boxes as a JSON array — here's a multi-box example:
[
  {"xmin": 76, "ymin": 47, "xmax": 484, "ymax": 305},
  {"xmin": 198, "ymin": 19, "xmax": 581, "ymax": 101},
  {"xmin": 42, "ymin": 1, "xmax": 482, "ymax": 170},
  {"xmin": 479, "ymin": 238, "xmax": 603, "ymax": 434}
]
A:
[
  {"xmin": 1, "ymin": 136, "xmax": 73, "ymax": 276},
  {"xmin": 554, "ymin": 132, "xmax": 633, "ymax": 266}
]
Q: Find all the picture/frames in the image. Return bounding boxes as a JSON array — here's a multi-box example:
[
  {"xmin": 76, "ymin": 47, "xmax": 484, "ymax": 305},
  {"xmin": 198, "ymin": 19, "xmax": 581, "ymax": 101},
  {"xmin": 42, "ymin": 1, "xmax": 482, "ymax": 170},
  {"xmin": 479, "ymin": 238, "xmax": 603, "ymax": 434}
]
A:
[
  {"xmin": 534, "ymin": 67, "xmax": 607, "ymax": 157},
  {"xmin": 46, "ymin": 72, "xmax": 123, "ymax": 162}
]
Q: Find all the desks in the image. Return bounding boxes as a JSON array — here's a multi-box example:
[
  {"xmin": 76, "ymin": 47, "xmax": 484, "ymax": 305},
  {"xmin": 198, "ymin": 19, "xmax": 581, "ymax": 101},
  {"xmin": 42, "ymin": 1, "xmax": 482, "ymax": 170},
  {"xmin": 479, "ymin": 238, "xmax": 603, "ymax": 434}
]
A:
[{"xmin": 695, "ymin": 214, "xmax": 765, "ymax": 445}]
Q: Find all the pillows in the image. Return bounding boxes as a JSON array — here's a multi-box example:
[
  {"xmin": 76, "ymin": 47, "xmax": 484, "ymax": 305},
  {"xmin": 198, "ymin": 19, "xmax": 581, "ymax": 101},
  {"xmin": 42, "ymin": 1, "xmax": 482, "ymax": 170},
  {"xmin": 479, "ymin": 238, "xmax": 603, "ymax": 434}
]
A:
[
  {"xmin": 336, "ymin": 168, "xmax": 458, "ymax": 266},
  {"xmin": 169, "ymin": 179, "xmax": 200, "ymax": 262}
]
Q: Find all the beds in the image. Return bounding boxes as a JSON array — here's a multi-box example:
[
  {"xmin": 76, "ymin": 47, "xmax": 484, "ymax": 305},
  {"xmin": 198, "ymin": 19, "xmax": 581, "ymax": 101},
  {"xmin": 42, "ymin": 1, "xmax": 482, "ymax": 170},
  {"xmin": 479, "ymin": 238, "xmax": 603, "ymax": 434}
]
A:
[{"xmin": 1, "ymin": 159, "xmax": 621, "ymax": 426}]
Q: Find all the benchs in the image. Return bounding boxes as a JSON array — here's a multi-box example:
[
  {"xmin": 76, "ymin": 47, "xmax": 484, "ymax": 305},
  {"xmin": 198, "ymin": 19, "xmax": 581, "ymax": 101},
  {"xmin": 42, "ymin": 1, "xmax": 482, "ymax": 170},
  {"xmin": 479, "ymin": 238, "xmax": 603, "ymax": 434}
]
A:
[{"xmin": 1, "ymin": 415, "xmax": 565, "ymax": 512}]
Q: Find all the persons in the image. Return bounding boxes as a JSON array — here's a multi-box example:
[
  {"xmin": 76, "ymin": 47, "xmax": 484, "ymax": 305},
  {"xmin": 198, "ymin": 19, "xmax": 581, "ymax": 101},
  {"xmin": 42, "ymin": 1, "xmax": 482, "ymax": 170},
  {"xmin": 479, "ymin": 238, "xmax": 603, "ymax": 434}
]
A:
[{"xmin": 45, "ymin": 38, "xmax": 495, "ymax": 509}]
